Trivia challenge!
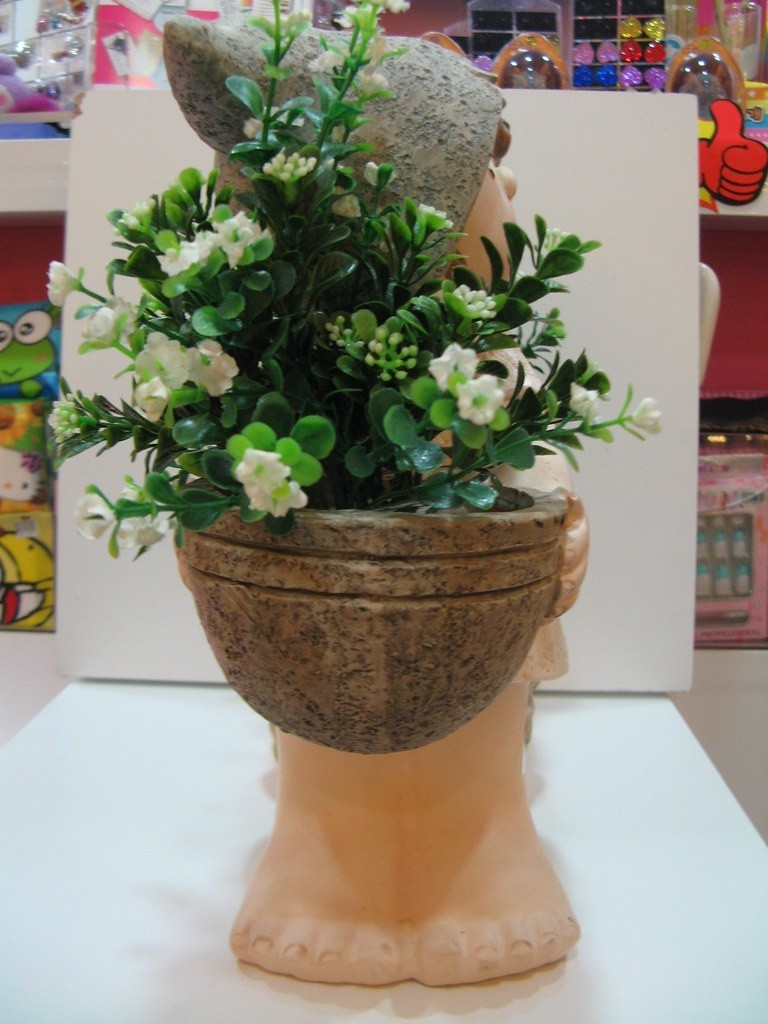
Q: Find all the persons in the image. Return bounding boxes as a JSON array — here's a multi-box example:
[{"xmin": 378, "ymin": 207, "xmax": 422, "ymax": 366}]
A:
[{"xmin": 165, "ymin": 18, "xmax": 589, "ymax": 984}]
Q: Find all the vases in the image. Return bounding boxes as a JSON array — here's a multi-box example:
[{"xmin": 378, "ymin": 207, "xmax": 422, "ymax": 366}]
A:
[{"xmin": 173, "ymin": 489, "xmax": 570, "ymax": 752}]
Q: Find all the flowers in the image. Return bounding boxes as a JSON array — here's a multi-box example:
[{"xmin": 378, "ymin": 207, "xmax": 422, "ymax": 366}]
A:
[{"xmin": 42, "ymin": 1, "xmax": 663, "ymax": 559}]
